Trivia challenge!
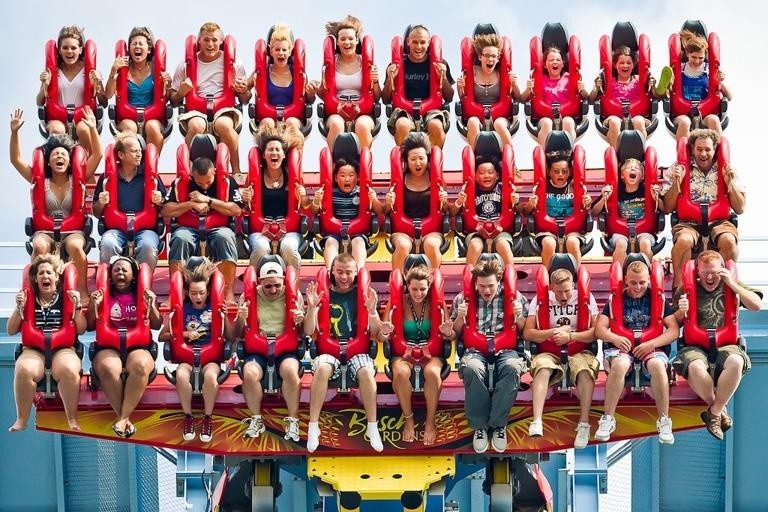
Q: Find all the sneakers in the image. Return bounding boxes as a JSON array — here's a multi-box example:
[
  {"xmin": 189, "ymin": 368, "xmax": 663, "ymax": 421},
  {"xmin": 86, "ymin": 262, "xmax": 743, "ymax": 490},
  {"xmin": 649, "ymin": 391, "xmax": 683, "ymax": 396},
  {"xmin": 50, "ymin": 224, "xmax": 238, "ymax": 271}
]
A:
[
  {"xmin": 198, "ymin": 414, "xmax": 213, "ymax": 442},
  {"xmin": 472, "ymin": 424, "xmax": 490, "ymax": 454},
  {"xmin": 528, "ymin": 421, "xmax": 544, "ymax": 437},
  {"xmin": 283, "ymin": 417, "xmax": 300, "ymax": 442},
  {"xmin": 574, "ymin": 422, "xmax": 591, "ymax": 449},
  {"xmin": 655, "ymin": 412, "xmax": 674, "ymax": 445},
  {"xmin": 594, "ymin": 414, "xmax": 616, "ymax": 441},
  {"xmin": 655, "ymin": 66, "xmax": 672, "ymax": 95},
  {"xmin": 183, "ymin": 413, "xmax": 196, "ymax": 441},
  {"xmin": 241, "ymin": 415, "xmax": 266, "ymax": 438},
  {"xmin": 491, "ymin": 425, "xmax": 507, "ymax": 453}
]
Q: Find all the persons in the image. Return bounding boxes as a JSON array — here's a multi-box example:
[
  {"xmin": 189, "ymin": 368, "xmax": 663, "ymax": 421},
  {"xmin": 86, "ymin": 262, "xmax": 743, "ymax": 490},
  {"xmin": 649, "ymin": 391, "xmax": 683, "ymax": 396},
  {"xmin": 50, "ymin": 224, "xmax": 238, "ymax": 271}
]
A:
[
  {"xmin": 309, "ymin": 159, "xmax": 385, "ymax": 271},
  {"xmin": 234, "ymin": 254, "xmax": 306, "ymax": 441},
  {"xmin": 242, "ymin": 121, "xmax": 313, "ymax": 275},
  {"xmin": 667, "ymin": 29, "xmax": 733, "ymax": 142},
  {"xmin": 671, "ymin": 250, "xmax": 763, "ymax": 441},
  {"xmin": 303, "ymin": 252, "xmax": 384, "ymax": 453},
  {"xmin": 6, "ymin": 254, "xmax": 87, "ymax": 430},
  {"xmin": 381, "ymin": 25, "xmax": 456, "ymax": 149},
  {"xmin": 36, "ymin": 23, "xmax": 109, "ymax": 154},
  {"xmin": 377, "ymin": 264, "xmax": 453, "ymax": 445},
  {"xmin": 313, "ymin": 15, "xmax": 383, "ymax": 148},
  {"xmin": 590, "ymin": 156, "xmax": 667, "ymax": 263},
  {"xmin": 523, "ymin": 150, "xmax": 594, "ymax": 266},
  {"xmin": 158, "ymin": 256, "xmax": 235, "ymax": 442},
  {"xmin": 519, "ymin": 267, "xmax": 599, "ymax": 449},
  {"xmin": 168, "ymin": 22, "xmax": 251, "ymax": 184},
  {"xmin": 384, "ymin": 132, "xmax": 448, "ymax": 272},
  {"xmin": 450, "ymin": 260, "xmax": 530, "ymax": 454},
  {"xmin": 9, "ymin": 106, "xmax": 105, "ymax": 307},
  {"xmin": 235, "ymin": 25, "xmax": 318, "ymax": 129},
  {"xmin": 103, "ymin": 26, "xmax": 172, "ymax": 156},
  {"xmin": 520, "ymin": 47, "xmax": 587, "ymax": 147},
  {"xmin": 161, "ymin": 158, "xmax": 241, "ymax": 267},
  {"xmin": 588, "ymin": 45, "xmax": 657, "ymax": 147},
  {"xmin": 592, "ymin": 260, "xmax": 681, "ymax": 444},
  {"xmin": 458, "ymin": 34, "xmax": 521, "ymax": 150},
  {"xmin": 664, "ymin": 128, "xmax": 747, "ymax": 291},
  {"xmin": 92, "ymin": 130, "xmax": 166, "ymax": 278},
  {"xmin": 448, "ymin": 153, "xmax": 520, "ymax": 266},
  {"xmin": 84, "ymin": 258, "xmax": 164, "ymax": 435}
]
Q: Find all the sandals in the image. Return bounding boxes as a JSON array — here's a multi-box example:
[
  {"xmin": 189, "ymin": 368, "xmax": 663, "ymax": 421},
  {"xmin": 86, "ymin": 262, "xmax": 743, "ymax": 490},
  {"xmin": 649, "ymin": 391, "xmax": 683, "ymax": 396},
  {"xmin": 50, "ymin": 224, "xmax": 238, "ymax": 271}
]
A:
[
  {"xmin": 111, "ymin": 424, "xmax": 124, "ymax": 437},
  {"xmin": 365, "ymin": 429, "xmax": 384, "ymax": 452},
  {"xmin": 307, "ymin": 426, "xmax": 321, "ymax": 453},
  {"xmin": 721, "ymin": 408, "xmax": 733, "ymax": 433},
  {"xmin": 700, "ymin": 406, "xmax": 723, "ymax": 440},
  {"xmin": 125, "ymin": 424, "xmax": 137, "ymax": 439}
]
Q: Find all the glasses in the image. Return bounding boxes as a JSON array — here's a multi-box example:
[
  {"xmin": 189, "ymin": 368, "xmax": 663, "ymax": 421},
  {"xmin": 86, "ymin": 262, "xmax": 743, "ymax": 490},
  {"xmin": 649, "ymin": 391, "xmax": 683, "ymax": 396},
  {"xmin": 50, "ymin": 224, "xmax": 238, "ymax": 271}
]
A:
[
  {"xmin": 481, "ymin": 52, "xmax": 500, "ymax": 59},
  {"xmin": 262, "ymin": 283, "xmax": 281, "ymax": 289},
  {"xmin": 550, "ymin": 167, "xmax": 568, "ymax": 175}
]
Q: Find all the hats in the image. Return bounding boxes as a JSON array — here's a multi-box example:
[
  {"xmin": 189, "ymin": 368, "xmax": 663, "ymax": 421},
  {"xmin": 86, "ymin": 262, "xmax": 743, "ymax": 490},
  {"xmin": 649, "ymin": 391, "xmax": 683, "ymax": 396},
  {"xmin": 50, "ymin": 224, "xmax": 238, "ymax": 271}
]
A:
[{"xmin": 259, "ymin": 261, "xmax": 285, "ymax": 279}]
